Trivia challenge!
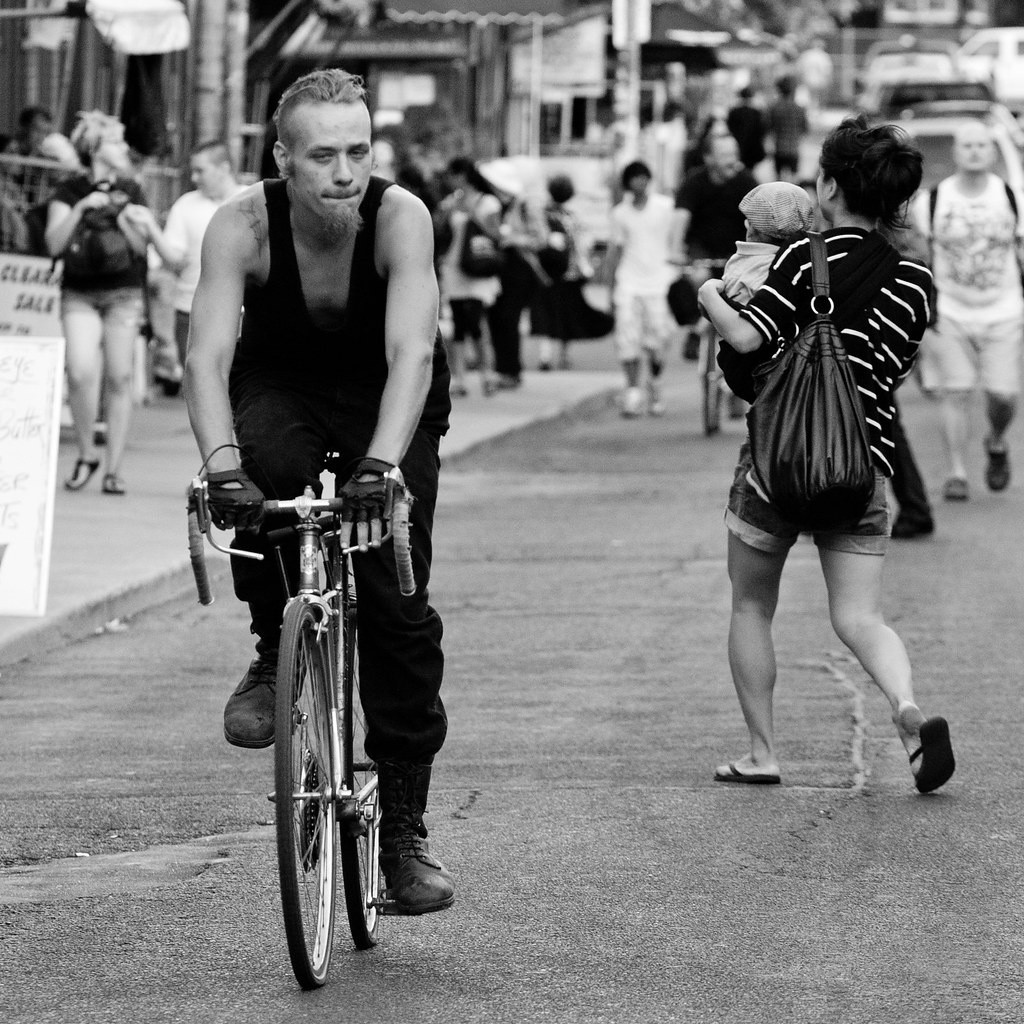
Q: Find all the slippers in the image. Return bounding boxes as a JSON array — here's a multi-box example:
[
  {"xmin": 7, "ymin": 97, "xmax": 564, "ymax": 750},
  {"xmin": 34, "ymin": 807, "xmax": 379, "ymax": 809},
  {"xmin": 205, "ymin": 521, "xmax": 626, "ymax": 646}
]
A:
[
  {"xmin": 714, "ymin": 763, "xmax": 780, "ymax": 783},
  {"xmin": 908, "ymin": 717, "xmax": 955, "ymax": 793}
]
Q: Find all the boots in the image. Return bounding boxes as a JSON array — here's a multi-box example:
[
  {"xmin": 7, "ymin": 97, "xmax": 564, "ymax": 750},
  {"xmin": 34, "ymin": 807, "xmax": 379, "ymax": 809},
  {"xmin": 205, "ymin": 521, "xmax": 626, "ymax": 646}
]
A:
[
  {"xmin": 224, "ymin": 599, "xmax": 306, "ymax": 748},
  {"xmin": 378, "ymin": 759, "xmax": 455, "ymax": 914}
]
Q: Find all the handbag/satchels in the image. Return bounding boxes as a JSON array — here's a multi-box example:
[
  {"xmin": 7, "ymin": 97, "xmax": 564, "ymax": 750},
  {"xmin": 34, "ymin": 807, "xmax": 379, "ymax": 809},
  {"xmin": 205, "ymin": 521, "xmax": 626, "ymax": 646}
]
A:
[{"xmin": 746, "ymin": 317, "xmax": 874, "ymax": 529}]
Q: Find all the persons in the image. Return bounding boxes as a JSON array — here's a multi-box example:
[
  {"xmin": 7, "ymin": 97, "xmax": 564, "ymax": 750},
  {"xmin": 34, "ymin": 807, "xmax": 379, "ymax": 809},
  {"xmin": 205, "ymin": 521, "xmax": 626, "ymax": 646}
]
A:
[
  {"xmin": 908, "ymin": 115, "xmax": 1024, "ymax": 502},
  {"xmin": 646, "ymin": 77, "xmax": 810, "ymax": 195},
  {"xmin": 368, "ymin": 131, "xmax": 614, "ymax": 389},
  {"xmin": 0, "ymin": 105, "xmax": 253, "ymax": 494},
  {"xmin": 599, "ymin": 164, "xmax": 680, "ymax": 417},
  {"xmin": 714, "ymin": 180, "xmax": 813, "ymax": 402},
  {"xmin": 184, "ymin": 69, "xmax": 456, "ymax": 915},
  {"xmin": 667, "ymin": 133, "xmax": 758, "ymax": 361},
  {"xmin": 695, "ymin": 116, "xmax": 958, "ymax": 794}
]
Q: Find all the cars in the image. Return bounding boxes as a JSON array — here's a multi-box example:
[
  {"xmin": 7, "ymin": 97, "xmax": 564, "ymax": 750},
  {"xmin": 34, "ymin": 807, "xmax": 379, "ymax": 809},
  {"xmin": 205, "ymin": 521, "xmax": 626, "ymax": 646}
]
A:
[{"xmin": 866, "ymin": 24, "xmax": 1024, "ymax": 197}]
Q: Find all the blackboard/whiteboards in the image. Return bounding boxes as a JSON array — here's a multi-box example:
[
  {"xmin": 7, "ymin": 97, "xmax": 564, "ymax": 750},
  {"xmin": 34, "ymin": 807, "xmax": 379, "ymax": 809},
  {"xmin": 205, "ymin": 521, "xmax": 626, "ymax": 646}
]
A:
[
  {"xmin": 0, "ymin": 252, "xmax": 62, "ymax": 338},
  {"xmin": 0, "ymin": 338, "xmax": 66, "ymax": 615}
]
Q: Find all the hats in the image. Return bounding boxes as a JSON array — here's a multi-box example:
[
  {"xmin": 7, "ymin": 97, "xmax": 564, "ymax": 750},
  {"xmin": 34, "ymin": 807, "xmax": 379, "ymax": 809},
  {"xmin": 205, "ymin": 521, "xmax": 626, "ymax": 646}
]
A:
[{"xmin": 738, "ymin": 181, "xmax": 814, "ymax": 240}]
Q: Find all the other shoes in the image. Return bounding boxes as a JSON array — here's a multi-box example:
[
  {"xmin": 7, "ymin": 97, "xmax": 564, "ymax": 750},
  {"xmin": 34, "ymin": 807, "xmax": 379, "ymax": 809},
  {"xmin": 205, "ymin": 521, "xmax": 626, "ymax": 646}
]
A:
[
  {"xmin": 944, "ymin": 476, "xmax": 969, "ymax": 499},
  {"xmin": 65, "ymin": 460, "xmax": 99, "ymax": 490},
  {"xmin": 683, "ymin": 333, "xmax": 701, "ymax": 359},
  {"xmin": 649, "ymin": 379, "xmax": 668, "ymax": 415},
  {"xmin": 621, "ymin": 387, "xmax": 641, "ymax": 415},
  {"xmin": 892, "ymin": 511, "xmax": 932, "ymax": 538},
  {"xmin": 984, "ymin": 435, "xmax": 1010, "ymax": 489},
  {"xmin": 103, "ymin": 474, "xmax": 125, "ymax": 493}
]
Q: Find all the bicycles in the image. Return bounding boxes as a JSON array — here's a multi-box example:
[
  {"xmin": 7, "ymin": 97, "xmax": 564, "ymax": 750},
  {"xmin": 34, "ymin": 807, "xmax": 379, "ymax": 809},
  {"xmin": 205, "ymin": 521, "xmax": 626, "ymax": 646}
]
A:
[
  {"xmin": 668, "ymin": 257, "xmax": 746, "ymax": 435},
  {"xmin": 186, "ymin": 444, "xmax": 417, "ymax": 990}
]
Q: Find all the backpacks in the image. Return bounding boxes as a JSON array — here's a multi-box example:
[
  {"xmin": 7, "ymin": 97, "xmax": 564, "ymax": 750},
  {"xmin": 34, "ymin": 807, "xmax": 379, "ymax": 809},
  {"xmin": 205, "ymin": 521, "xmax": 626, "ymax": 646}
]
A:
[{"xmin": 62, "ymin": 179, "xmax": 133, "ymax": 293}]
